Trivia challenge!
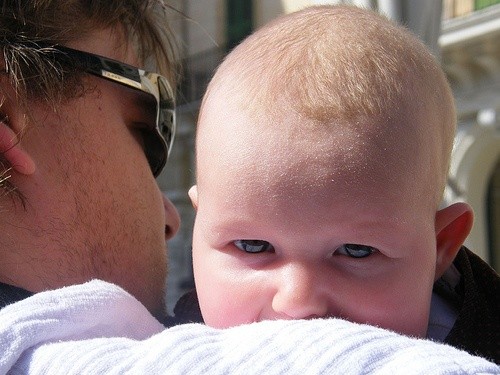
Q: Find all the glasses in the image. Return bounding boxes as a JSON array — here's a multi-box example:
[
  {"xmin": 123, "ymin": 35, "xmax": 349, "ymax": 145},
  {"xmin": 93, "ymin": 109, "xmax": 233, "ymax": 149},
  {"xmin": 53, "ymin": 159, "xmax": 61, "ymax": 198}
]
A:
[{"xmin": 0, "ymin": 35, "xmax": 176, "ymax": 178}]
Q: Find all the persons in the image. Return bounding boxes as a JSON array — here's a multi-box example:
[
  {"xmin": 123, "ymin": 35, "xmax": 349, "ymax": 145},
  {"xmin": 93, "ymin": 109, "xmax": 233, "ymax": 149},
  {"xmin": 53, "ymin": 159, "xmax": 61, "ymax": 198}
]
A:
[
  {"xmin": 0, "ymin": 0, "xmax": 500, "ymax": 374},
  {"xmin": 173, "ymin": 2, "xmax": 499, "ymax": 366}
]
